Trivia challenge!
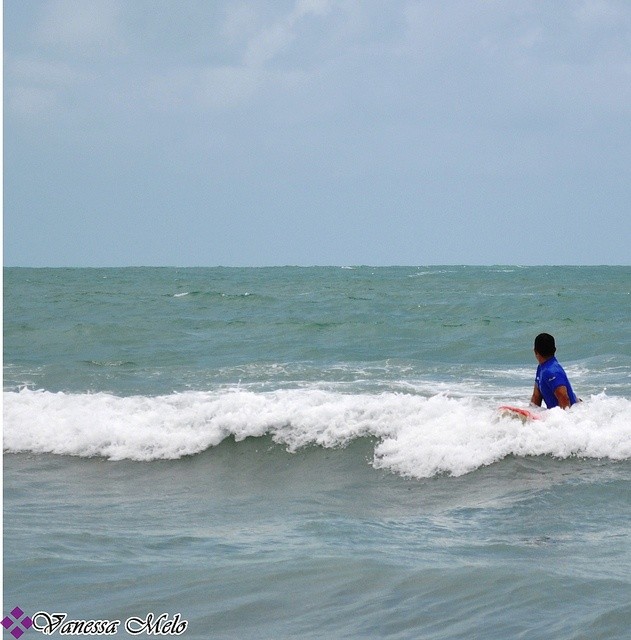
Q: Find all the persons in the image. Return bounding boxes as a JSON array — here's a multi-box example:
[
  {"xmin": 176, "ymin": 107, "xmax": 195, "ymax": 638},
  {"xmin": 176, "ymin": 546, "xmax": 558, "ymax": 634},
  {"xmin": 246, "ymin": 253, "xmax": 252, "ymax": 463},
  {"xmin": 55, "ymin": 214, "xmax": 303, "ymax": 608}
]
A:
[{"xmin": 528, "ymin": 333, "xmax": 586, "ymax": 412}]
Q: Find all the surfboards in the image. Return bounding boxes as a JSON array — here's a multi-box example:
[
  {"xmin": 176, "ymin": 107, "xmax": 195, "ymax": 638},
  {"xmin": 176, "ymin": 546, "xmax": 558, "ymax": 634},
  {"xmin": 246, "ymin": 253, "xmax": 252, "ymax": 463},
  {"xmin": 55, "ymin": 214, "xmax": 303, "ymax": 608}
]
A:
[{"xmin": 498, "ymin": 405, "xmax": 545, "ymax": 420}]
